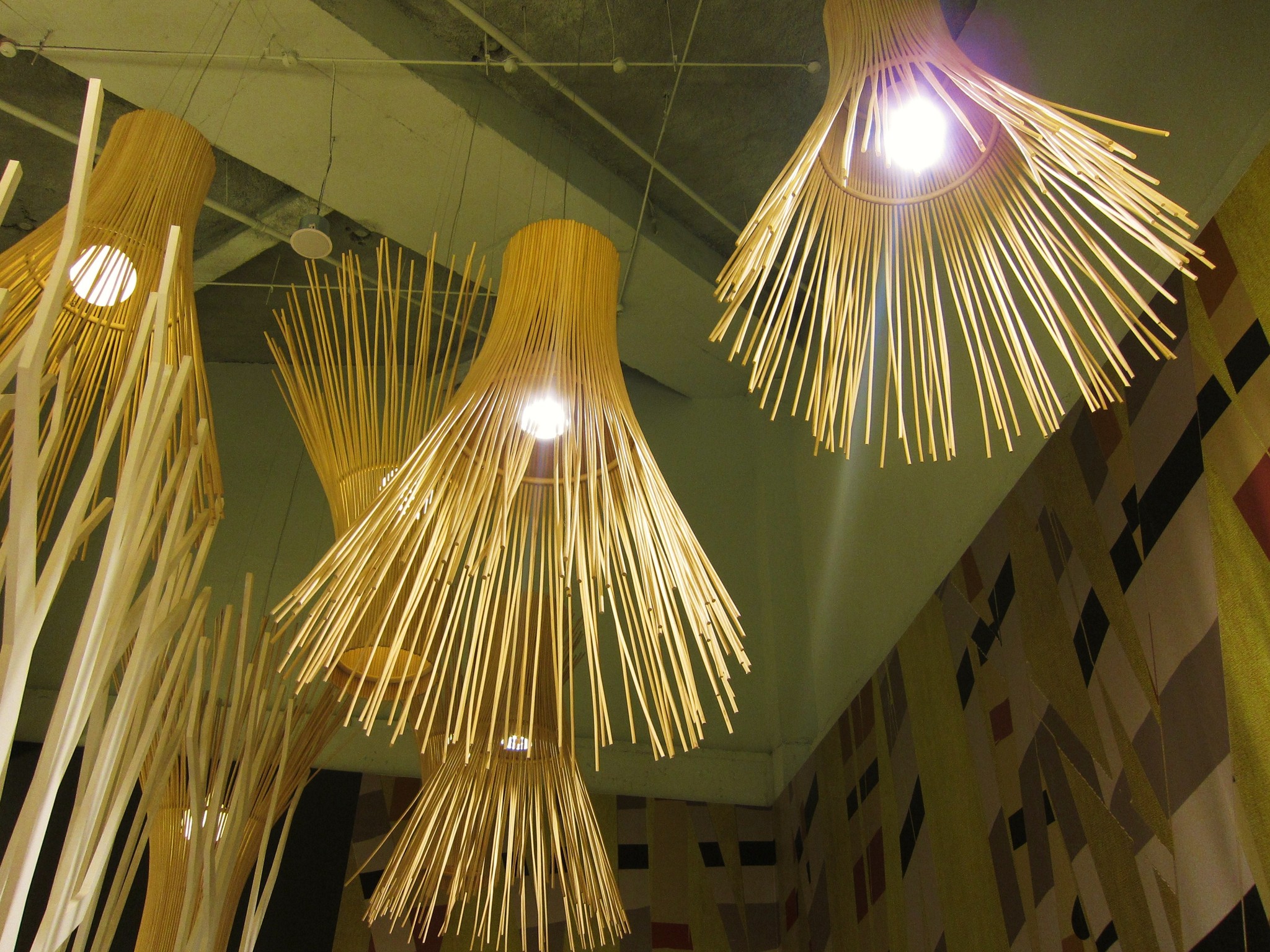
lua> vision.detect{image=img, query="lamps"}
[289,57,335,259]
[341,586,634,951]
[709,1,1214,466]
[259,1,502,714]
[0,1,256,566]
[268,1,746,761]
[112,446,359,952]
